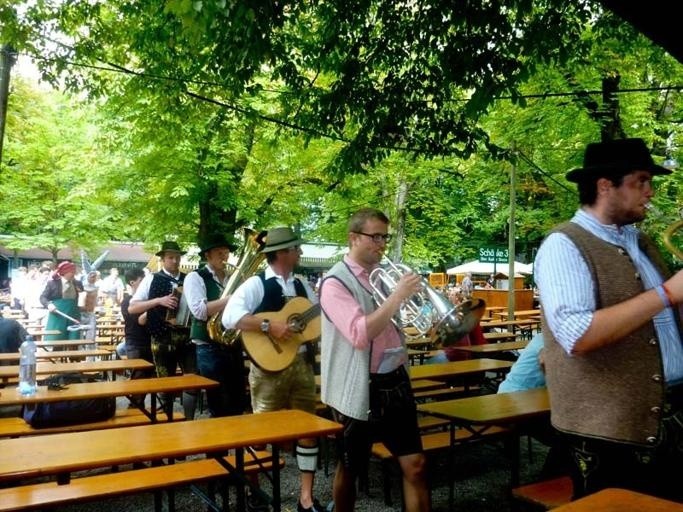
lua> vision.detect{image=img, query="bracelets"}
[654,286,670,308]
[660,284,674,306]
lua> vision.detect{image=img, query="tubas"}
[369,248,484,349]
[207,227,265,345]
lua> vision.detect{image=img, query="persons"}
[533,139,683,503]
[496,334,579,482]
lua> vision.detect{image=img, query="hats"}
[258,227,305,254]
[155,242,187,256]
[564,138,672,181]
[198,235,238,256]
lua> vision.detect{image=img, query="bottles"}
[17,335,40,396]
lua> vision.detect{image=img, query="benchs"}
[511,476,574,510]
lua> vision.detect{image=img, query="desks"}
[2,306,345,510]
[551,487,682,511]
[371,287,551,510]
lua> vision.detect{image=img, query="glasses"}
[351,231,392,244]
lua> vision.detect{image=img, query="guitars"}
[242,297,323,372]
